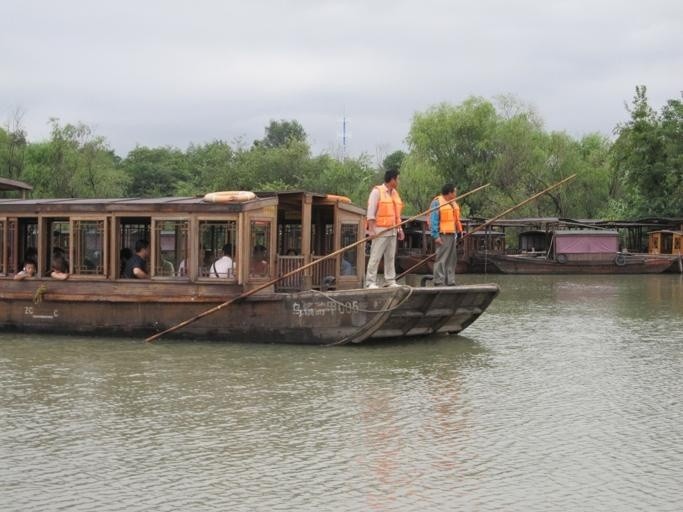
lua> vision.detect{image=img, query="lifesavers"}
[203,190,256,203]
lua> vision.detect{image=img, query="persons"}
[426,182,465,288]
[358,169,405,288]
[178,243,236,279]
[120,240,175,278]
[250,245,352,277]
[13,247,101,281]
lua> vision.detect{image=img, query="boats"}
[0,190,501,344]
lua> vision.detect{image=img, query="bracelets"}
[397,228,403,232]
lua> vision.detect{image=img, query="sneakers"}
[366,284,377,289]
[388,284,399,287]
